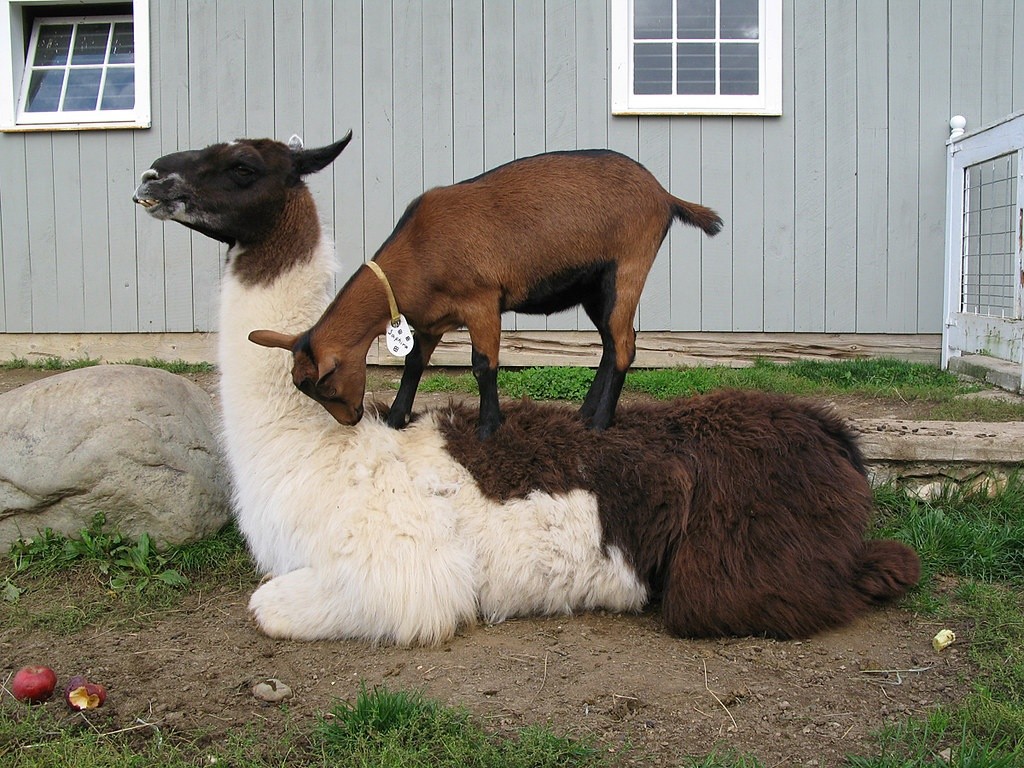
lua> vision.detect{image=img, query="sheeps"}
[248,149,726,437]
[133,129,921,646]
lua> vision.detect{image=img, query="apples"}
[65,677,105,710]
[13,666,57,704]
